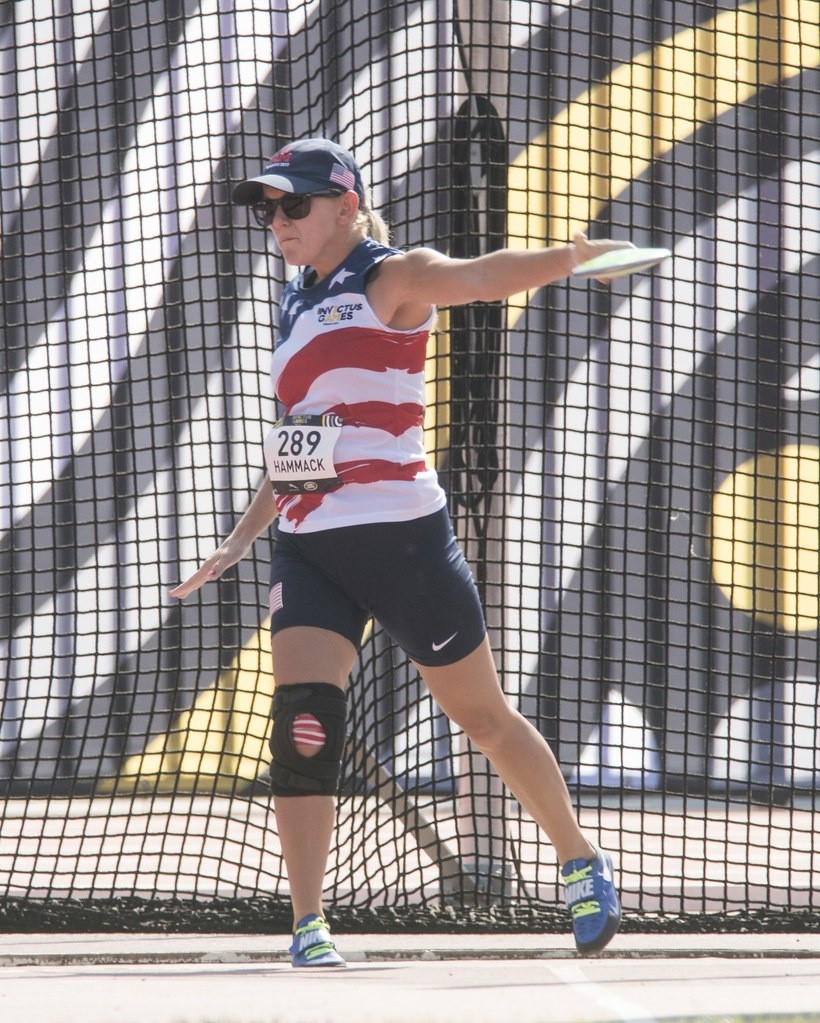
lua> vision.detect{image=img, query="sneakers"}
[290,913,348,968]
[560,837,621,955]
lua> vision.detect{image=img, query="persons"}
[169,137,638,968]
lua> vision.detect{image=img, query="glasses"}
[252,188,344,227]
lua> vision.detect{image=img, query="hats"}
[230,139,363,210]
